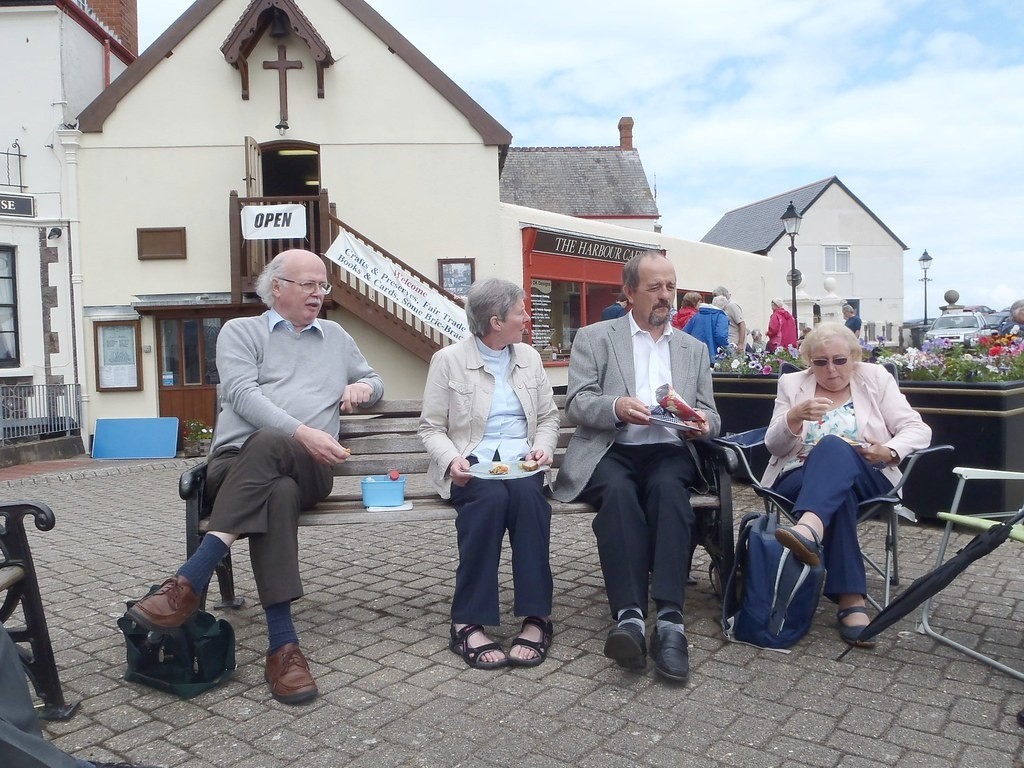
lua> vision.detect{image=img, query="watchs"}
[889,448,896,464]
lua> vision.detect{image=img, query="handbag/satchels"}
[117,585,237,700]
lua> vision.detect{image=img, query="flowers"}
[183,416,204,440]
[711,322,1024,384]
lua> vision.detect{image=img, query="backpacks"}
[720,513,826,651]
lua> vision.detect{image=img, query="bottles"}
[384,470,400,481]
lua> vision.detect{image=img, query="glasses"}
[274,275,333,297]
[809,356,849,366]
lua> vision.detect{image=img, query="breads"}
[522,460,537,471]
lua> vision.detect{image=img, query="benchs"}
[179,394,739,609]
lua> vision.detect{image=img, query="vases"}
[710,372,1024,535]
[184,440,212,456]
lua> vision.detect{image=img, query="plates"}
[648,416,702,432]
[800,440,868,448]
[463,460,543,480]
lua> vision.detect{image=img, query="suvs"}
[964,305,996,315]
[923,311,996,347]
[982,312,1011,331]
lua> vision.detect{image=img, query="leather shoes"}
[265,641,318,704]
[650,624,690,681]
[126,576,203,633]
[603,624,647,669]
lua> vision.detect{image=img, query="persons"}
[760,321,932,646]
[877,337,885,348]
[799,327,812,340]
[129,249,384,704]
[561,310,581,351]
[541,250,722,683]
[670,286,754,368]
[1002,307,1024,332]
[601,294,630,321]
[842,305,861,339]
[765,297,798,355]
[751,329,766,352]
[417,278,561,670]
[0,622,161,768]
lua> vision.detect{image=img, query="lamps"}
[48,228,62,240]
[275,115,289,137]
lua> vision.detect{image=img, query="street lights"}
[780,200,804,341]
[917,248,933,326]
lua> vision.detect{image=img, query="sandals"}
[508,617,553,666]
[448,621,507,669]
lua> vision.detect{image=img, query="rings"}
[627,409,634,415]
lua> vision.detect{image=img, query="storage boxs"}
[720,427,772,485]
[361,475,405,507]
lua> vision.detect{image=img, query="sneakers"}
[775,523,823,566]
[837,606,877,645]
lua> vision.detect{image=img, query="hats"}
[772,298,783,307]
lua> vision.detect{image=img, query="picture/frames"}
[438,257,476,288]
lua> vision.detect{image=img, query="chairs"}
[0,501,82,719]
[922,467,1024,681]
[712,361,956,612]
